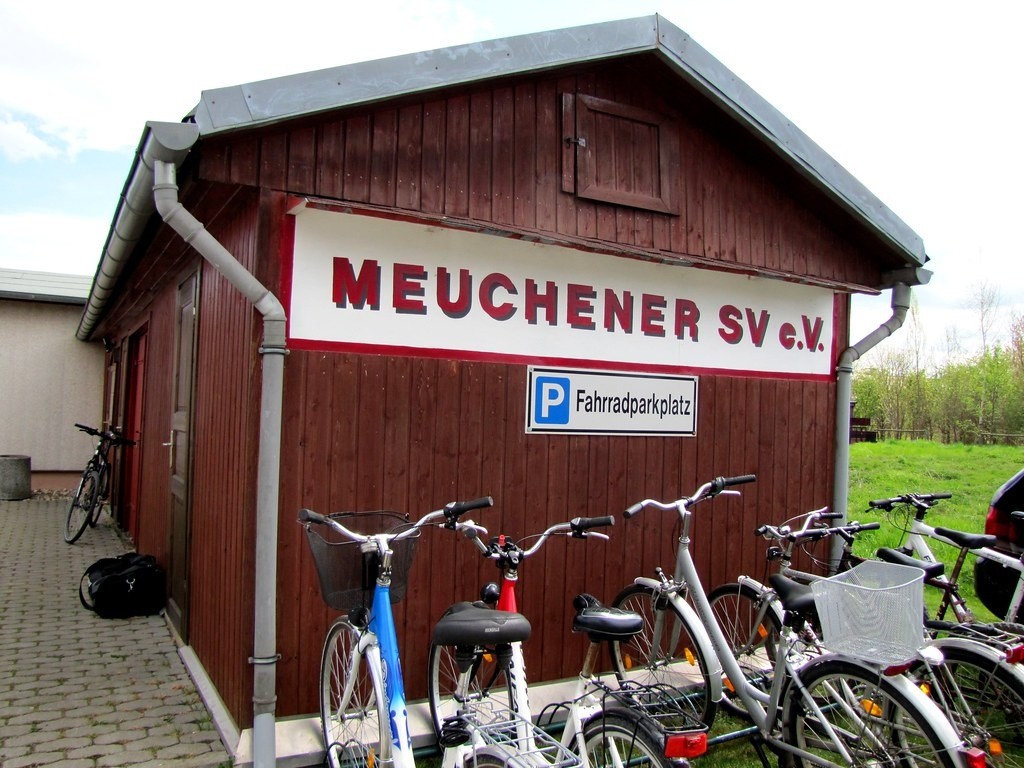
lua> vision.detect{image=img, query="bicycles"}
[296,493,581,768]
[63,422,138,545]
[426,513,710,768]
[705,488,1023,767]
[607,473,988,768]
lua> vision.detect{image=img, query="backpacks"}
[78,553,165,617]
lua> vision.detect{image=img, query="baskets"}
[303,512,420,611]
[810,561,925,666]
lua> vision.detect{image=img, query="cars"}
[974,466,1024,627]
